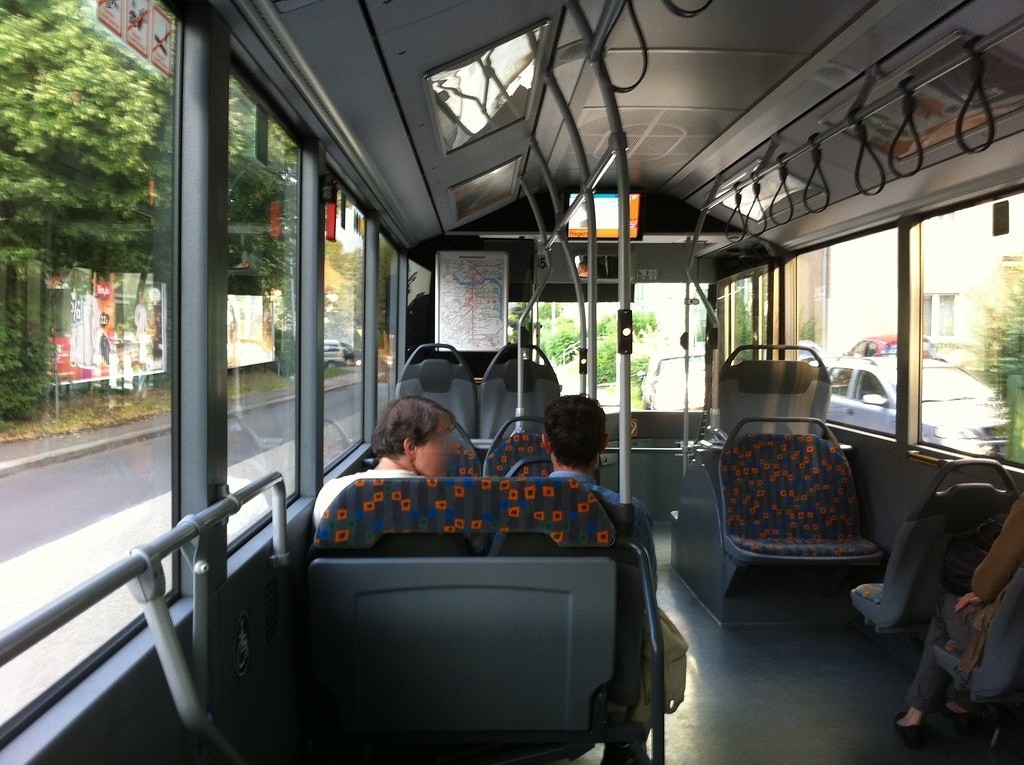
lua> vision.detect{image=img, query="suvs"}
[819,356,1013,460]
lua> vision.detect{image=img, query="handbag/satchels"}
[940,518,1002,595]
[642,603,689,715]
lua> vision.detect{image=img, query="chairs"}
[697,345,832,449]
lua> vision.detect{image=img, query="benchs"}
[720,418,884,570]
[851,458,1023,708]
[303,343,670,765]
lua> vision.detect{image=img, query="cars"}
[323,340,354,370]
[638,352,706,411]
[797,340,825,363]
[834,335,940,371]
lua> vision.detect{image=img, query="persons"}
[541,393,658,594]
[311,398,462,537]
[894,490,1024,749]
[578,262,588,278]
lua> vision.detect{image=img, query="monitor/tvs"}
[567,193,644,242]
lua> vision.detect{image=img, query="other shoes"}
[600,744,637,765]
[894,712,923,749]
[940,701,975,735]
